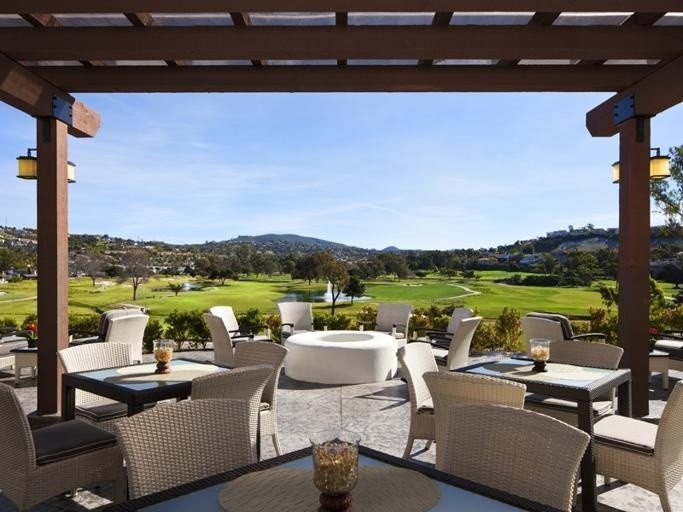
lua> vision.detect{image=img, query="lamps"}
[15,147,36,182]
[65,158,78,186]
[648,145,672,182]
[611,157,621,185]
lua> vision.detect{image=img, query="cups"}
[529,337,550,361]
[309,428,362,497]
[151,338,173,363]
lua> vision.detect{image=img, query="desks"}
[64,437,562,512]
[59,353,234,423]
[447,355,634,512]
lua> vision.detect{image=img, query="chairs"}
[416,368,526,423]
[434,400,594,512]
[232,338,291,460]
[199,298,486,376]
[523,338,625,488]
[393,342,443,462]
[188,363,276,463]
[521,292,682,424]
[53,340,156,432]
[0,303,150,388]
[0,381,118,512]
[112,396,252,499]
[592,377,683,512]
